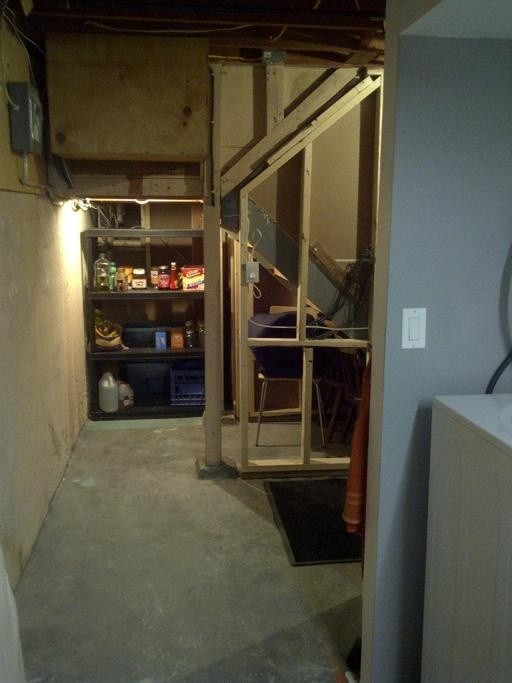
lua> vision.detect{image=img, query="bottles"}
[93,254,109,289]
[159,266,170,288]
[116,263,132,290]
[184,321,196,347]
[110,262,116,289]
[170,262,179,289]
[119,380,135,408]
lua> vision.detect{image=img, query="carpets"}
[264,478,361,567]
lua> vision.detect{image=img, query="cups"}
[149,267,159,286]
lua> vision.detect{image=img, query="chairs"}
[249,309,339,449]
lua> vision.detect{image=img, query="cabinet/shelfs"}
[85,229,206,422]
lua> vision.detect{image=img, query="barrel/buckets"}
[98,371,119,413]
[93,252,112,291]
[117,381,136,409]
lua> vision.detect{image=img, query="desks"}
[421,394,511,683]
